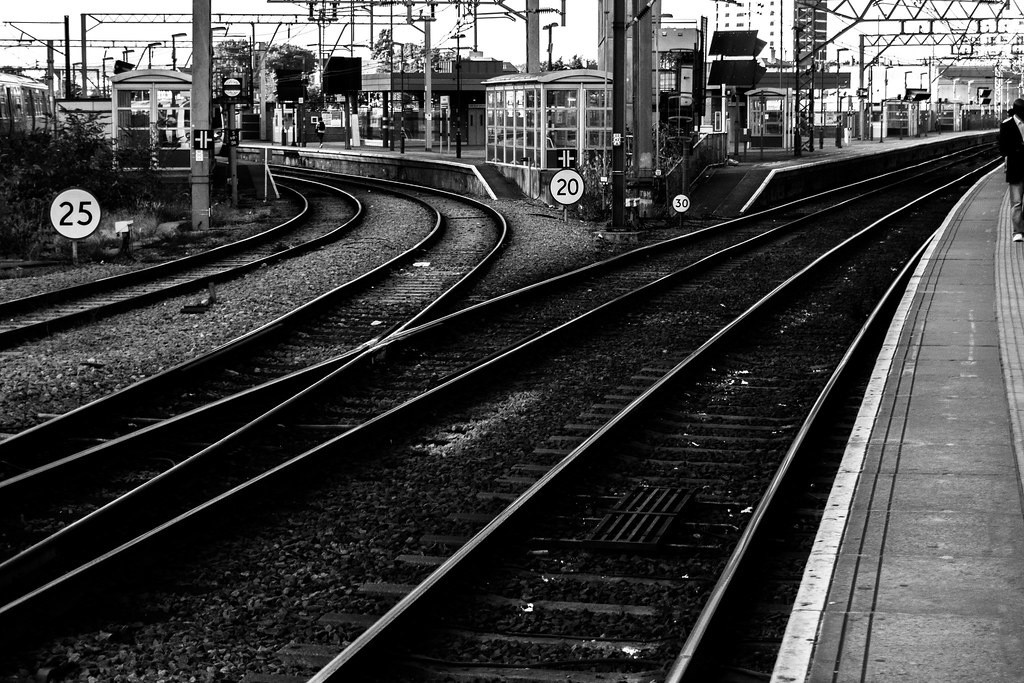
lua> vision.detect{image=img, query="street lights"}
[72,62,83,97]
[148,43,161,69]
[172,33,188,70]
[102,57,114,98]
[123,50,135,61]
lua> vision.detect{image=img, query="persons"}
[1002,109,1014,173]
[315,117,325,148]
[1001,98,1024,241]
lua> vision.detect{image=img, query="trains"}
[0,72,53,136]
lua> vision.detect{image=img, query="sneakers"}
[1011,234,1024,242]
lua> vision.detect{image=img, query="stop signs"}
[222,78,243,99]
